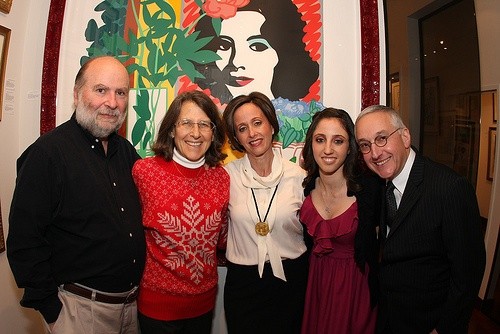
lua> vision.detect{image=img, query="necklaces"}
[318,179,330,211]
[250,185,279,237]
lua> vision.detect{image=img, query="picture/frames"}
[422,77,440,133]
[487,127,497,180]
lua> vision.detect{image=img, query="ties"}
[384,180,398,230]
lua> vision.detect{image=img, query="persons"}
[221,91,308,334]
[348,102,500,334]
[132,89,231,334]
[7,56,147,334]
[298,108,377,334]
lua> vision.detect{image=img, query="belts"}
[63,284,139,305]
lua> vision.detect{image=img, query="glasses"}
[359,128,400,154]
[175,118,216,132]
